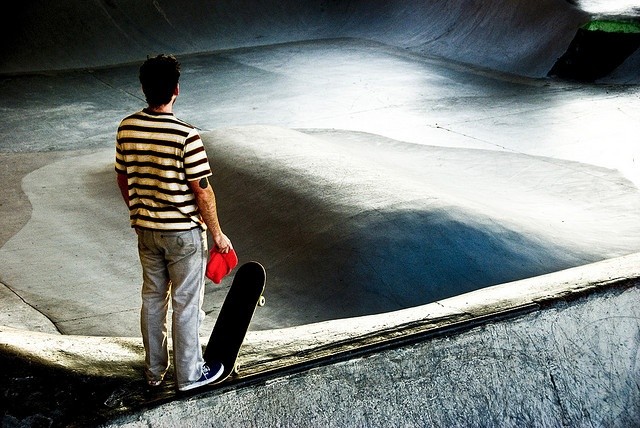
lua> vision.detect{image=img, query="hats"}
[205,242,238,284]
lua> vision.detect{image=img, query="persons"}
[114,52,233,394]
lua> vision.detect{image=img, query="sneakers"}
[177,360,225,390]
[148,365,170,386]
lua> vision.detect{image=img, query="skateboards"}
[203,262,267,386]
